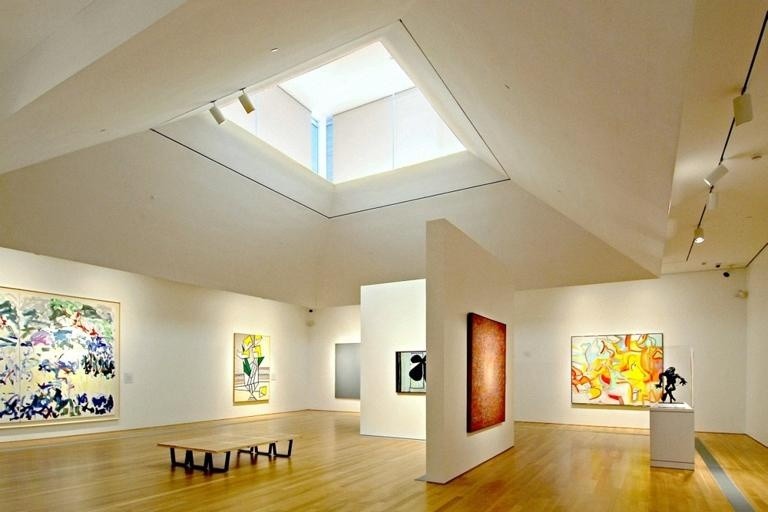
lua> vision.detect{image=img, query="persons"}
[655,367,688,401]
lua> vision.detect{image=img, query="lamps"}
[208,99,225,124]
[237,87,256,114]
[686,10,768,260]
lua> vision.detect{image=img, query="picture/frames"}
[0,285,122,429]
[467,311,506,433]
[395,351,427,393]
[571,333,664,407]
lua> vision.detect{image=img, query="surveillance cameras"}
[309,309,313,312]
[723,272,729,277]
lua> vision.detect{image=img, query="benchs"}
[157,433,296,476]
